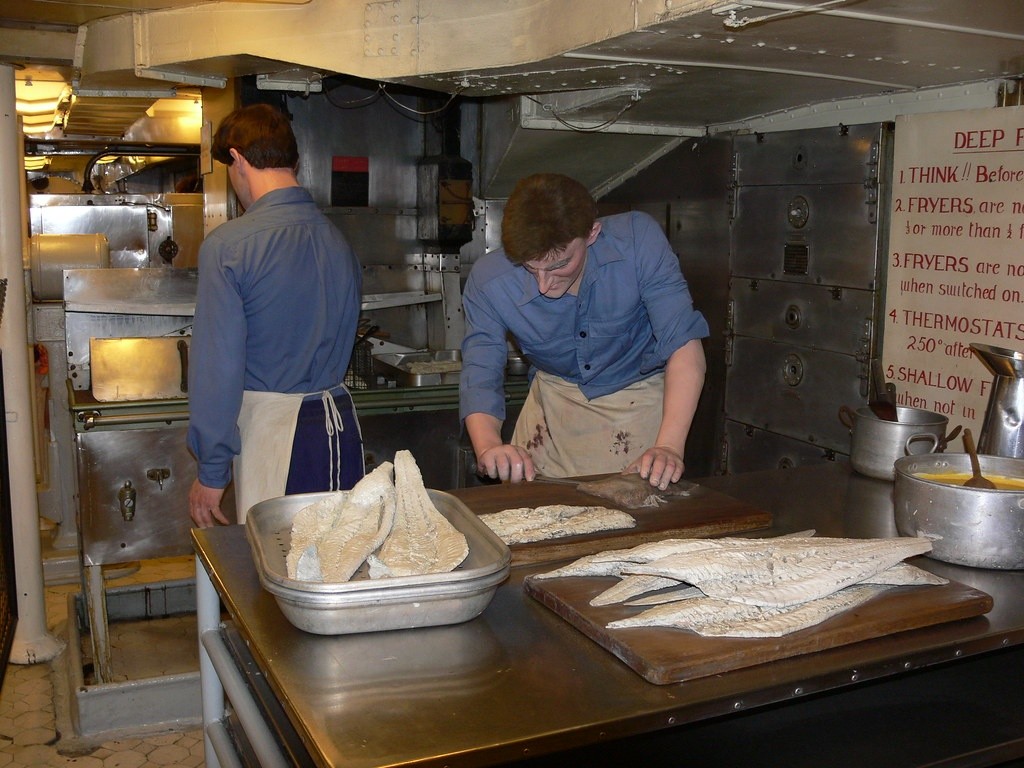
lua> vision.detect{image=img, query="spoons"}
[886,382,897,422]
[871,357,898,423]
[964,428,997,491]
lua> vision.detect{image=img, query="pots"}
[892,433,1024,571]
[837,402,962,482]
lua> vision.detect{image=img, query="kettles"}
[968,340,1024,460]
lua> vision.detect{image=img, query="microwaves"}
[61,311,196,409]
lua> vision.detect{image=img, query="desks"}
[188,458,1024,767]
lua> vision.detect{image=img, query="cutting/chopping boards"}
[444,470,771,567]
[521,563,992,688]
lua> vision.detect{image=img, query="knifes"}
[481,466,585,486]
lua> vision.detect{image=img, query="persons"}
[185,102,369,527]
[456,173,710,490]
